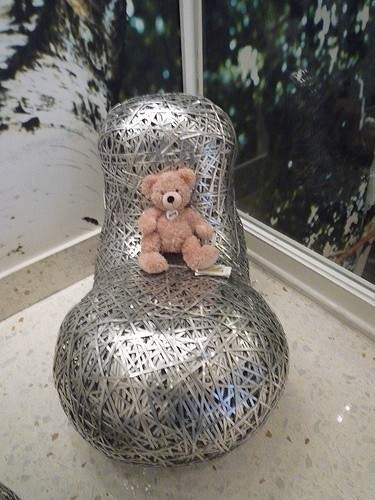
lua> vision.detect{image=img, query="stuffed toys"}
[135,166,219,276]
